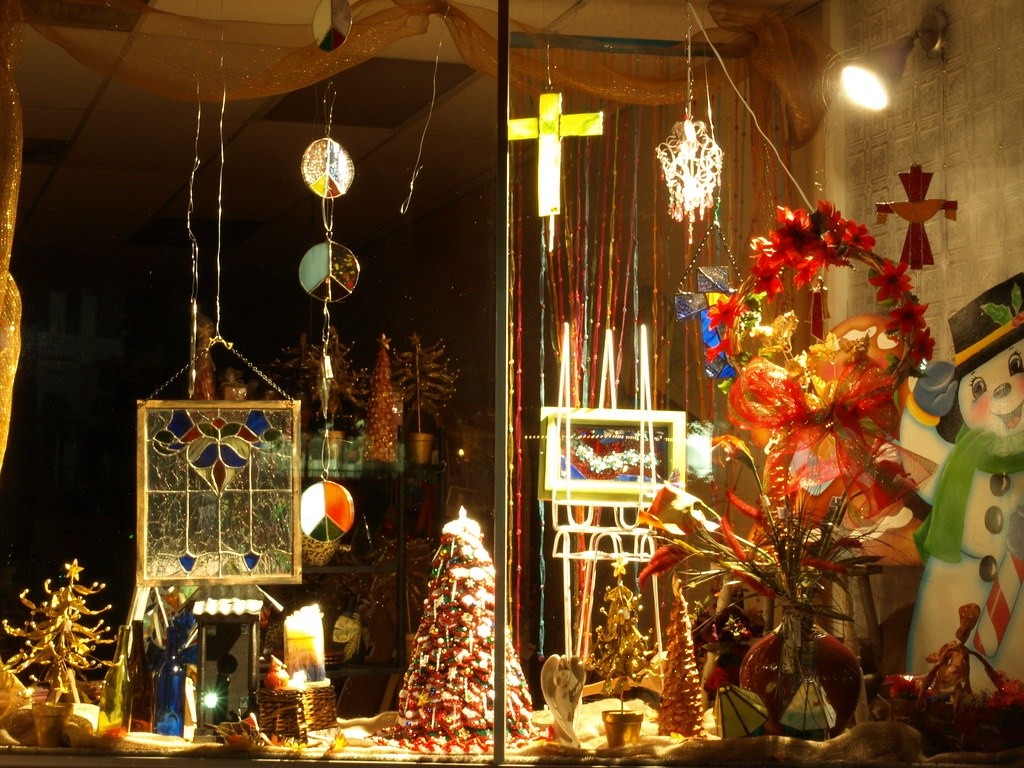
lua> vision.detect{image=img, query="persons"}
[148,585,199,741]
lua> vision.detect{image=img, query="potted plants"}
[587,574,671,749]
[7,562,126,750]
[300,325,371,474]
[392,332,461,472]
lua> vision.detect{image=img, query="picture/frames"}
[538,406,686,509]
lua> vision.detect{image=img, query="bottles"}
[265,657,289,691]
[129,619,155,732]
[98,625,130,734]
[157,627,185,736]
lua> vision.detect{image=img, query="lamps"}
[654,0,725,248]
[839,8,947,112]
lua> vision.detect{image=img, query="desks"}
[700,515,928,729]
